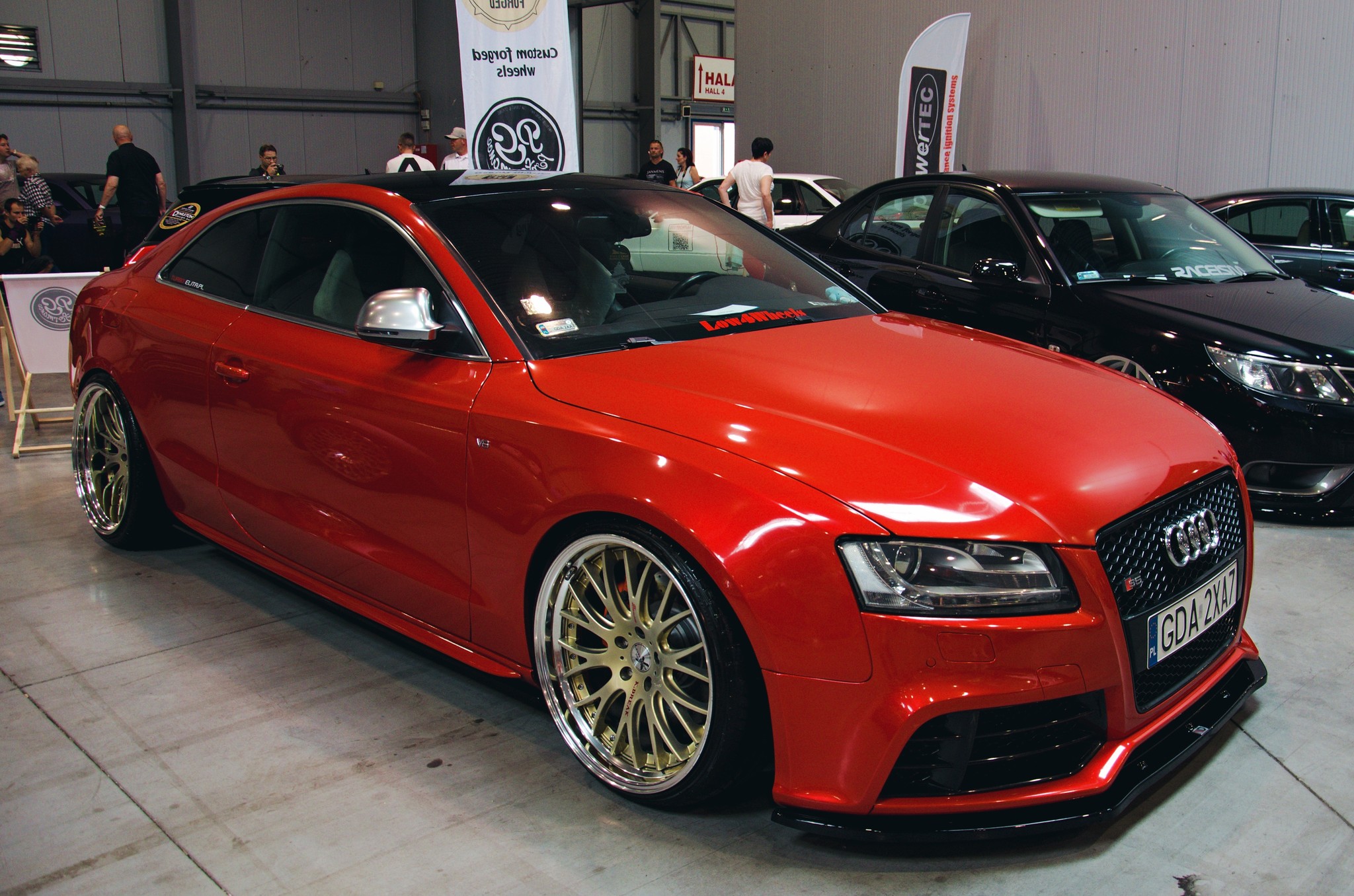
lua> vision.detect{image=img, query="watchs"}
[98,207,100,209]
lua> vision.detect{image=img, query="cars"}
[775,171,1354,520]
[64,171,1271,833]
[1092,186,1354,293]
[687,172,861,230]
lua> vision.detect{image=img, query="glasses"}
[263,157,277,161]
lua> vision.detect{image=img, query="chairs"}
[1297,222,1312,245]
[1050,218,1105,277]
[947,208,1027,275]
[311,252,368,327]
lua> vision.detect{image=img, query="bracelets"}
[263,174,265,176]
[99,205,105,209]
[33,232,40,237]
[266,171,270,176]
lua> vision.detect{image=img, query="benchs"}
[869,221,922,256]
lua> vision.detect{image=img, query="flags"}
[895,13,971,210]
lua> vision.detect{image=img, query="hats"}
[444,127,466,139]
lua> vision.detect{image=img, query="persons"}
[639,140,678,187]
[13,149,63,254]
[0,133,40,218]
[386,132,436,174]
[0,198,44,309]
[718,137,774,229]
[675,148,700,189]
[440,127,489,170]
[94,125,166,252]
[249,145,286,177]
